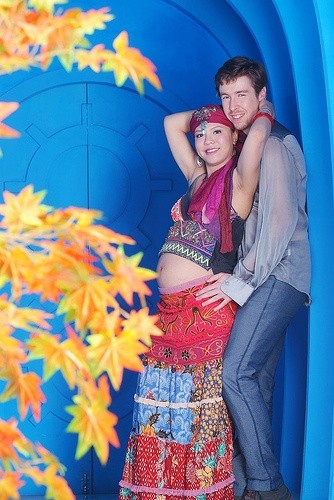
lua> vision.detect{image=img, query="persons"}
[195,56,312,500]
[118,101,275,500]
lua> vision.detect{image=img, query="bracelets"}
[251,102,274,128]
